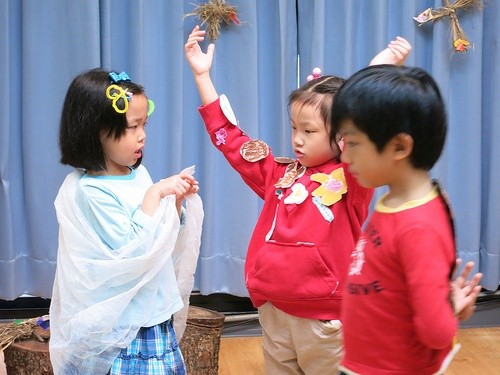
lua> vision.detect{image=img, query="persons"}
[329,64,482,375]
[49,70,204,375]
[184,25,412,375]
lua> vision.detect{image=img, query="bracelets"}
[158,189,165,200]
